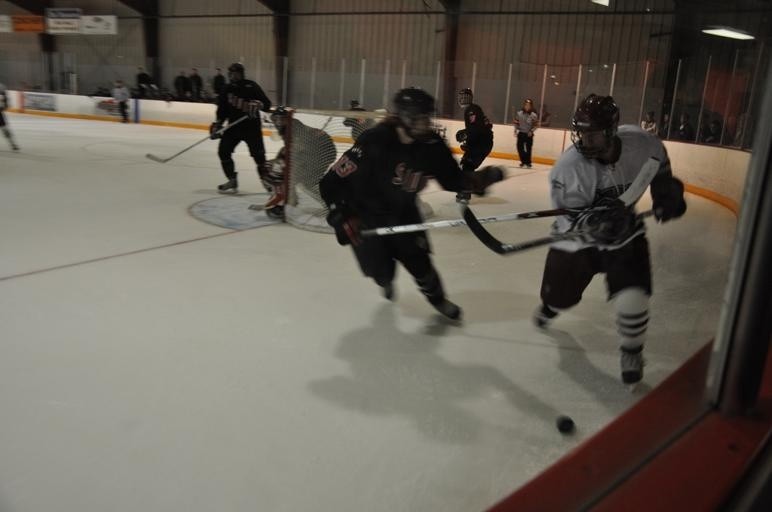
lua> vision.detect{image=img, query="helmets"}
[389,87,434,141]
[570,94,620,159]
[457,87,473,108]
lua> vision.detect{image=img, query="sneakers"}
[262,192,285,209]
[621,345,643,383]
[218,173,237,190]
[535,302,559,329]
[428,294,464,322]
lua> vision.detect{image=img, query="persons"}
[20,80,32,91]
[536,104,551,126]
[249,107,336,218]
[319,86,503,319]
[509,105,517,123]
[513,97,538,167]
[33,81,42,91]
[0,91,19,151]
[85,67,226,123]
[343,100,376,139]
[532,93,686,383]
[456,88,494,200]
[210,62,272,192]
[641,110,722,146]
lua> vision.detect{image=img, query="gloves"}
[569,196,637,244]
[456,129,470,142]
[209,122,224,140]
[244,100,265,118]
[650,174,687,224]
